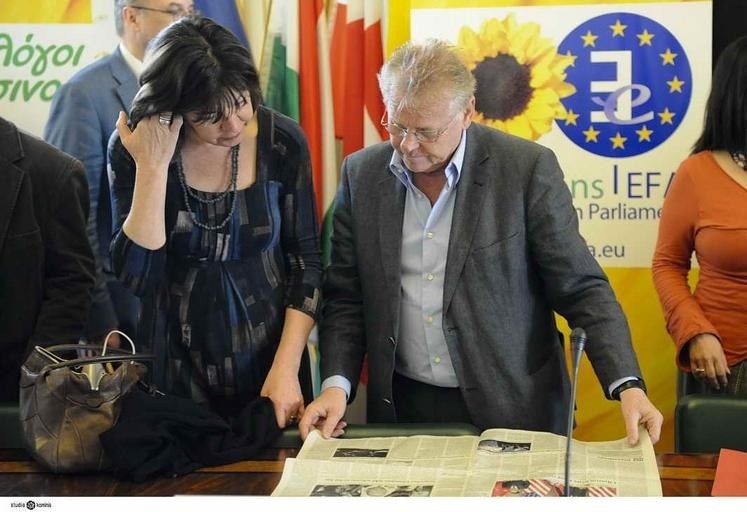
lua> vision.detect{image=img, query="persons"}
[298,37,666,450]
[651,35,747,398]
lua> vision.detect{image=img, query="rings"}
[696,368,705,372]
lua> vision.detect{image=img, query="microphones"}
[563,327,588,497]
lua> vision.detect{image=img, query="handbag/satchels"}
[17,342,158,478]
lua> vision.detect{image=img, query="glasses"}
[379,107,463,145]
[127,4,201,23]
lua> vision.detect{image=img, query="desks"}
[4,444,743,498]
